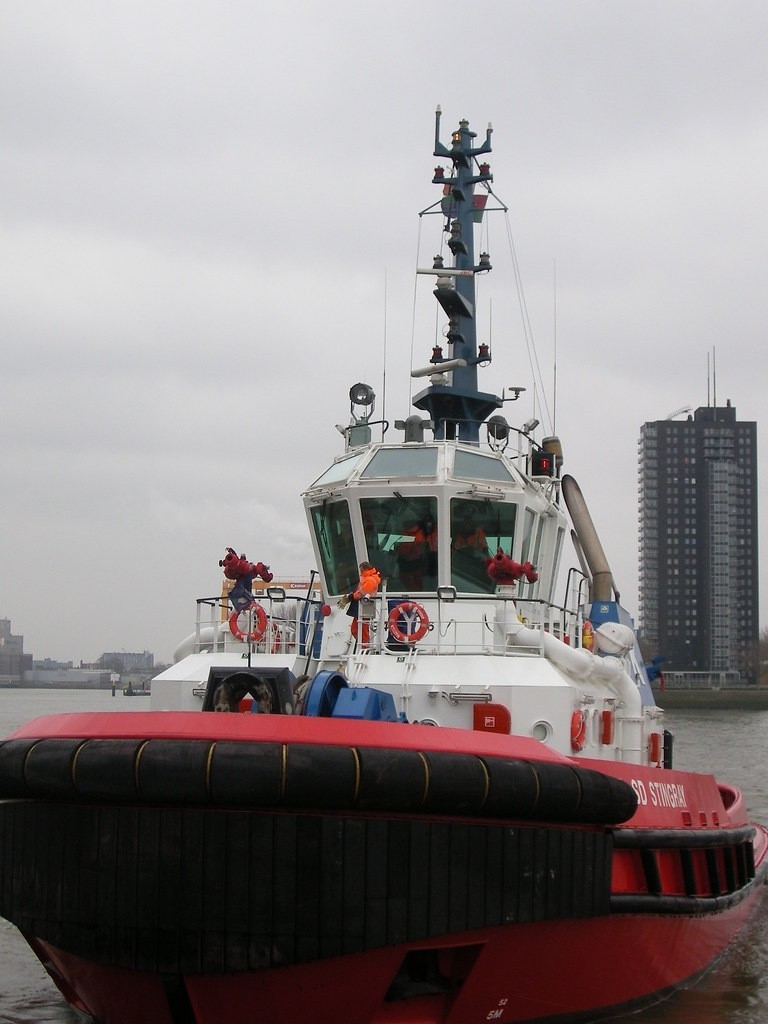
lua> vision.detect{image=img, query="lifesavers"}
[258,622,280,653]
[584,619,597,652]
[387,604,430,644]
[228,602,270,643]
[571,711,588,751]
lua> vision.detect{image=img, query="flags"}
[472,194,488,223]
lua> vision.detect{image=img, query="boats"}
[0,100,768,1023]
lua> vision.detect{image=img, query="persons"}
[415,514,438,551]
[451,517,488,554]
[337,562,381,655]
